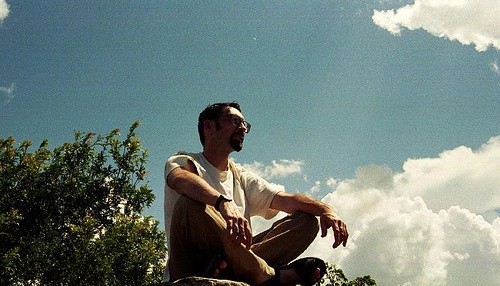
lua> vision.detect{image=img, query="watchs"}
[213,194,233,212]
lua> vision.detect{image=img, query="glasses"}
[213,115,251,133]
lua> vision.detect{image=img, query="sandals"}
[262,257,326,286]
[200,253,236,280]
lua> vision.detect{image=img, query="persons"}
[162,100,349,286]
